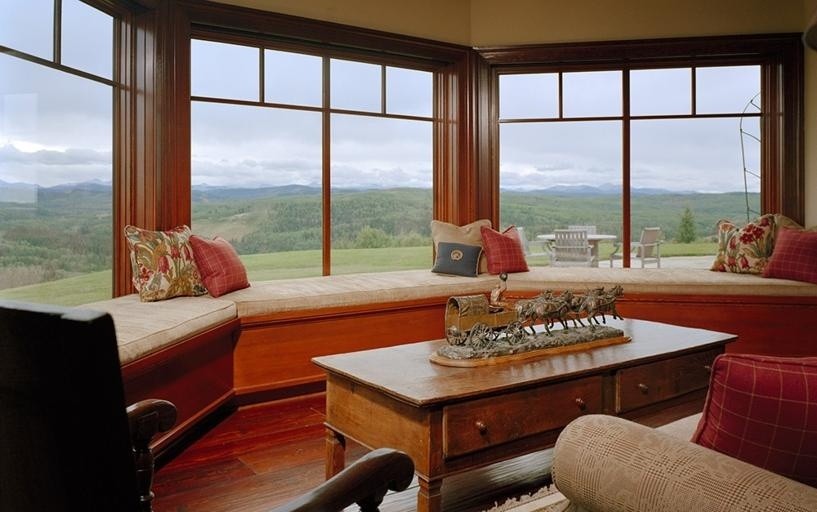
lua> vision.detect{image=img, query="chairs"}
[0,300,415,512]
[516,225,663,269]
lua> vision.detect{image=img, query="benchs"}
[500,266,817,357]
[219,269,500,396]
[73,293,240,460]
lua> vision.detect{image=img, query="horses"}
[514,285,624,337]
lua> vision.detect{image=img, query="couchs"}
[551,410,817,512]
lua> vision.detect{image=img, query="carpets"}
[483,484,571,512]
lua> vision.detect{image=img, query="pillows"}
[124,224,208,302]
[689,353,817,487]
[189,235,251,298]
[430,219,530,277]
[710,213,817,284]
